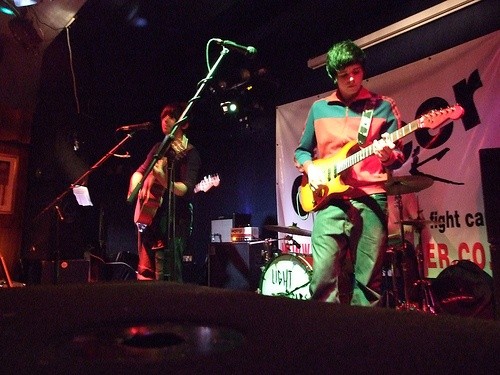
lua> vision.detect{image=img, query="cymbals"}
[392,217,446,228]
[383,175,434,195]
[265,224,309,236]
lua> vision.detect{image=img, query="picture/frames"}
[0,153,20,214]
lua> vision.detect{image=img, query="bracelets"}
[380,158,389,163]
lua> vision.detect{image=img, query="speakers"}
[209,242,274,294]
[479,147,500,323]
[39,259,91,285]
[211,213,251,243]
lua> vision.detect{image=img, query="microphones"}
[293,240,300,249]
[213,38,257,56]
[120,121,152,131]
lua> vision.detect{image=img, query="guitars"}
[193,173,221,194]
[299,102,465,213]
[132,133,190,226]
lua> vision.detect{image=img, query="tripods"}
[380,194,421,312]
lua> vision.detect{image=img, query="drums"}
[258,252,314,300]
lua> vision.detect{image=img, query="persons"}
[381,168,425,310]
[293,41,405,307]
[126,99,202,283]
